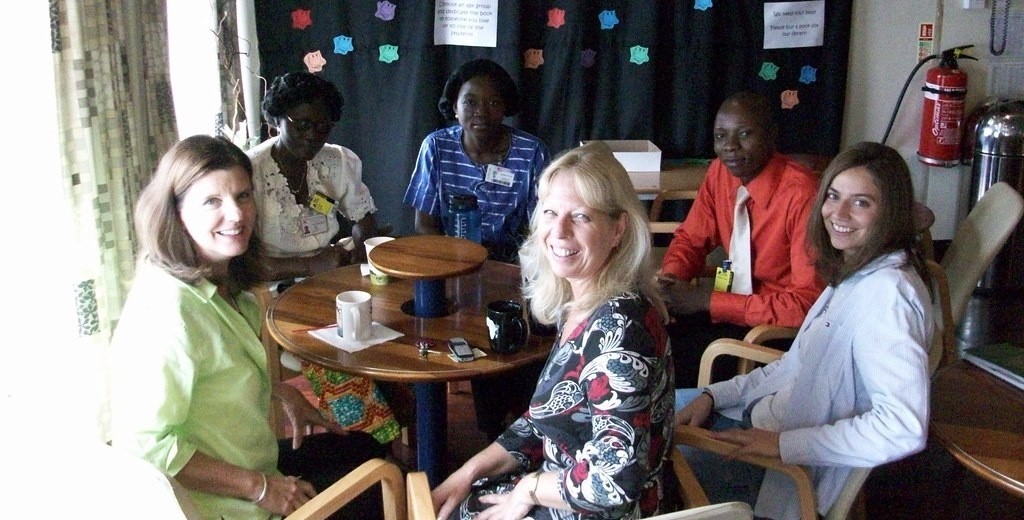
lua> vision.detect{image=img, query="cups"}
[527,299,558,335]
[484,302,529,353]
[448,194,481,248]
[336,290,372,342]
[363,237,397,286]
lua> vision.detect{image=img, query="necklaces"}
[274,147,305,194]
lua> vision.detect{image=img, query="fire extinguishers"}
[881,45,979,168]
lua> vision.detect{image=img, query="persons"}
[241,71,417,461]
[653,88,823,388]
[431,138,676,520]
[664,140,936,520]
[110,132,347,520]
[402,58,551,442]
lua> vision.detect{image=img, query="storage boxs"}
[578,139,662,172]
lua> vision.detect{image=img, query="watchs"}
[527,470,547,506]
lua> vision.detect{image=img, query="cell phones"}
[447,337,475,362]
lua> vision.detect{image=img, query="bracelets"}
[251,471,267,505]
[702,390,714,408]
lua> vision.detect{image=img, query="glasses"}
[285,114,335,134]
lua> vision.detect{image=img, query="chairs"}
[154,183,1024,520]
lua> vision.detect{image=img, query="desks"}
[622,166,710,237]
[266,235,560,484]
[931,355,1024,494]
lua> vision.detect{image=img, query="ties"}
[728,185,753,295]
